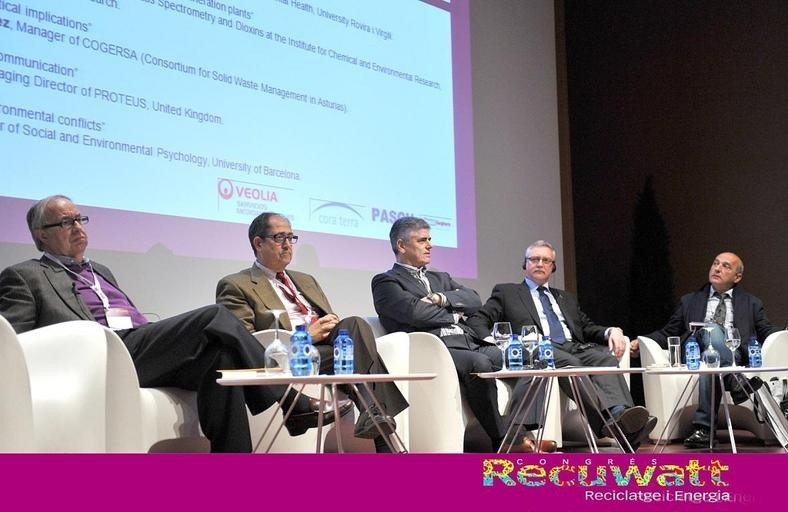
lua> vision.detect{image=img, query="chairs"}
[245,329,410,455]
[557,333,631,448]
[0,313,109,455]
[103,320,306,453]
[638,330,788,444]
[363,312,558,454]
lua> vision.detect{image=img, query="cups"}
[667,336,682,369]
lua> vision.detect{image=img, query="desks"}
[641,365,788,454]
[472,367,645,454]
[211,374,436,453]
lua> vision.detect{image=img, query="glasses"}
[258,233,298,245]
[41,216,89,228]
[525,257,553,264]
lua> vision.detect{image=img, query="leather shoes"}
[497,435,541,453]
[354,404,397,440]
[685,428,718,449]
[527,439,557,453]
[732,375,764,405]
[601,405,650,439]
[622,415,659,453]
[282,395,354,437]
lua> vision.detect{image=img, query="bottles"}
[684,337,700,370]
[537,335,555,368]
[333,328,354,375]
[748,334,762,368]
[289,317,313,376]
[508,334,524,370]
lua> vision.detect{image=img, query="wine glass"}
[493,321,513,372]
[263,308,291,377]
[702,325,721,368]
[723,327,744,369]
[520,325,539,370]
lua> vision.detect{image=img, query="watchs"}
[426,292,437,304]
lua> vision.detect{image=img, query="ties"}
[536,286,566,345]
[713,292,730,326]
[277,271,320,323]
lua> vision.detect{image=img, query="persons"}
[1,193,357,453]
[628,251,786,448]
[465,238,659,454]
[215,211,410,453]
[371,216,559,453]
